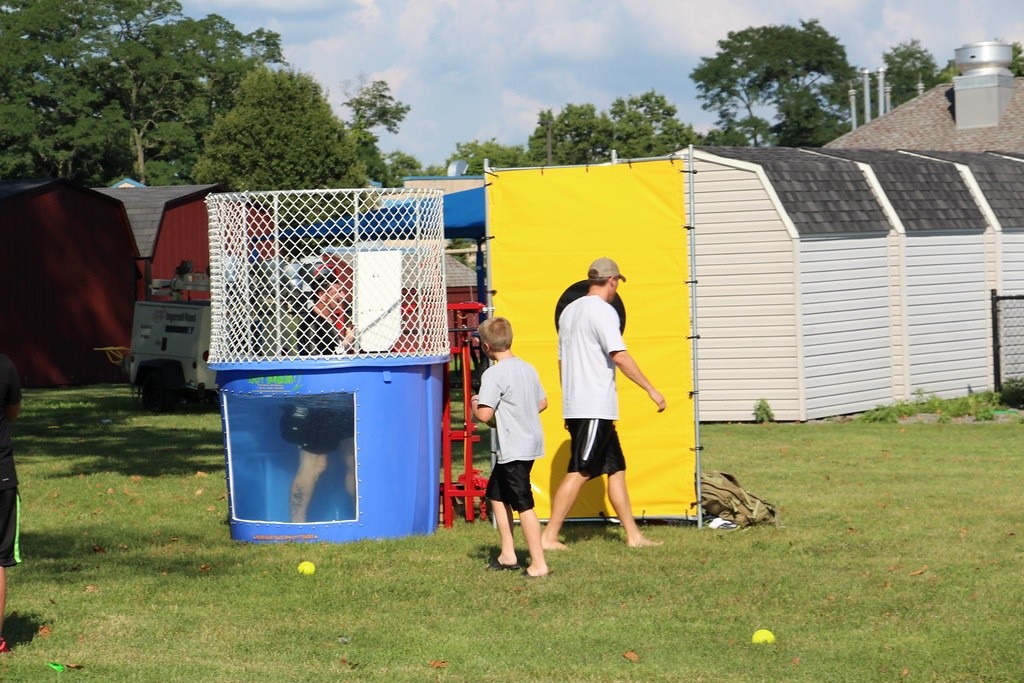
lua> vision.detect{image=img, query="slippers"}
[522,569,531,578]
[485,559,520,571]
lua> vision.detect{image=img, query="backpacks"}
[696,468,776,528]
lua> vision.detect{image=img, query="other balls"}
[752,629,775,645]
[298,561,316,576]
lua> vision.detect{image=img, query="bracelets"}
[473,399,478,401]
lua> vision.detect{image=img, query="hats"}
[588,258,626,282]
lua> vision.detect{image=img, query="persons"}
[289,274,356,355]
[0,352,23,654]
[542,256,667,550]
[471,316,554,579]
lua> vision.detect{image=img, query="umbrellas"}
[249,186,487,324]
